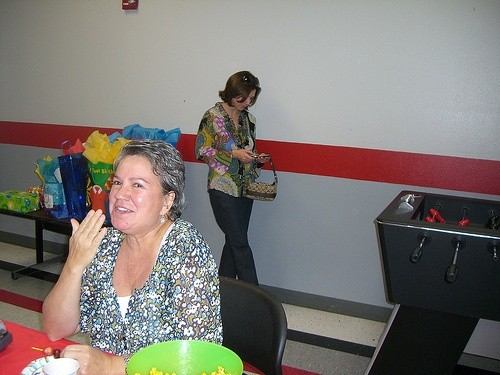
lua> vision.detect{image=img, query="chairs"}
[216,276,288,375]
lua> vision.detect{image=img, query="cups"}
[43,358,80,374]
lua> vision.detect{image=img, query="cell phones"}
[251,155,269,158]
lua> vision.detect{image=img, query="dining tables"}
[0,319,118,375]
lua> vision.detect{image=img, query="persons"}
[42,138,223,375]
[195,70,271,287]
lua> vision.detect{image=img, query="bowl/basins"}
[127,340,243,375]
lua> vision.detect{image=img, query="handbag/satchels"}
[242,151,278,202]
[41,152,117,224]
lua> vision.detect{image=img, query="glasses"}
[241,76,261,87]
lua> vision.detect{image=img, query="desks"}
[0,208,113,279]
